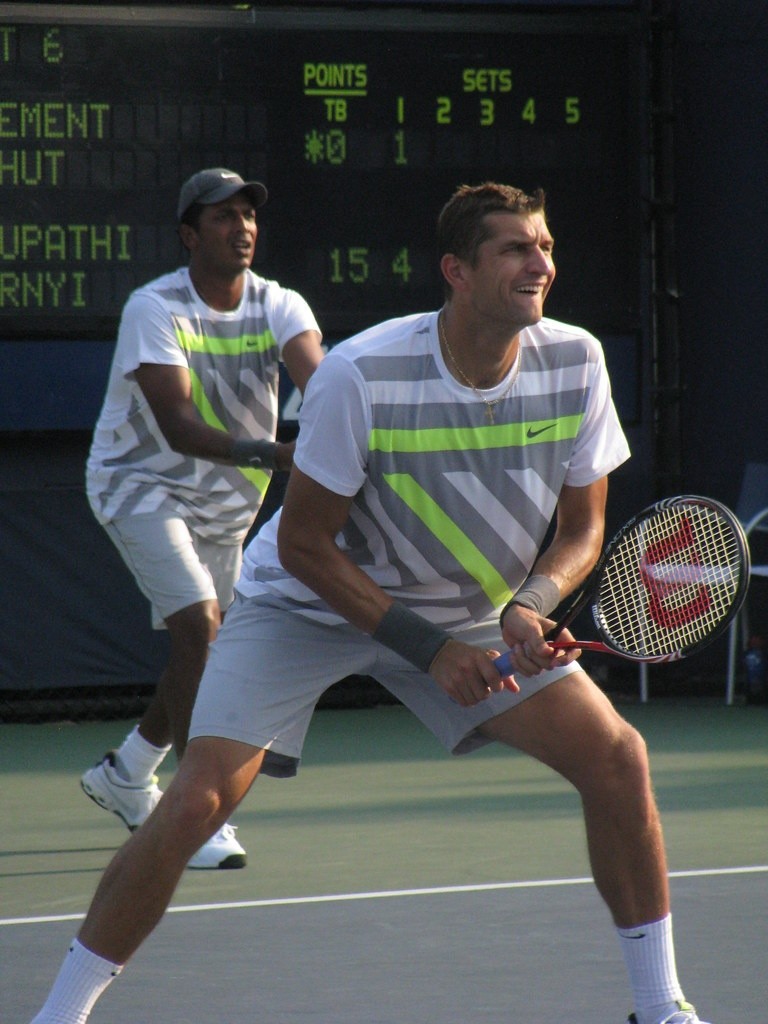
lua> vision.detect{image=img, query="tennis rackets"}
[447,493,751,706]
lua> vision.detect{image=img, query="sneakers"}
[186,822,247,869]
[80,752,164,836]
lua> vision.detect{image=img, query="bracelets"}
[230,439,282,472]
[372,600,453,673]
[499,574,560,631]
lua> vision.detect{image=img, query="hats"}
[176,167,269,224]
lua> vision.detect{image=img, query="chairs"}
[640,462,768,705]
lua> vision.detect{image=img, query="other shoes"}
[626,1000,711,1024]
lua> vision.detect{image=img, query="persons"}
[80,167,325,871]
[29,182,707,1023]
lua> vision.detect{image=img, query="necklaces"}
[439,315,521,426]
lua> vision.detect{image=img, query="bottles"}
[746,638,768,707]
